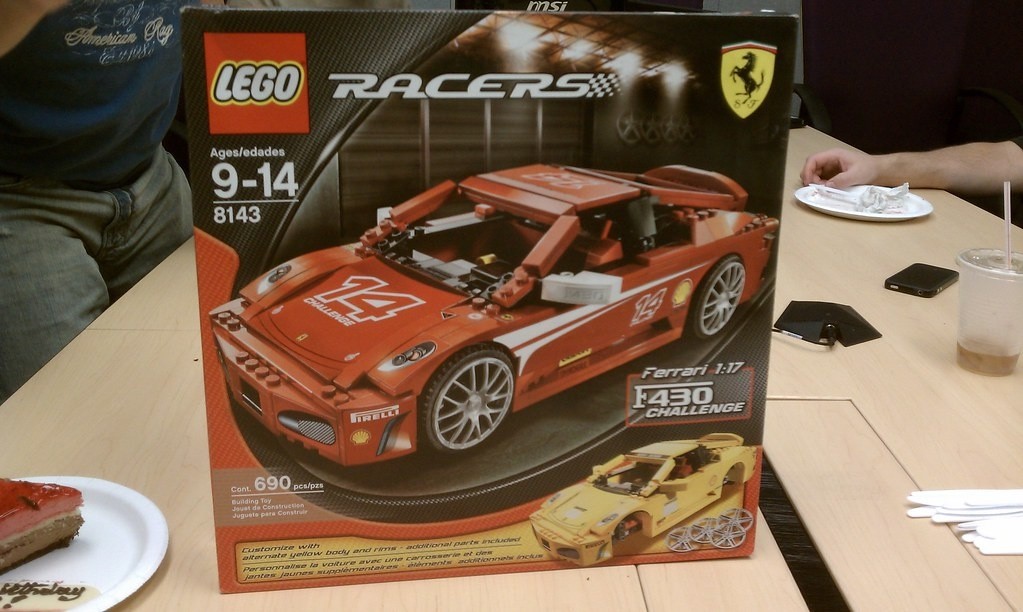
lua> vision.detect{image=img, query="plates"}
[795,184,934,223]
[0,476,168,612]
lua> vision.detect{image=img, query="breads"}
[0,582,103,612]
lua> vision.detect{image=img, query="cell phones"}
[886,263,959,298]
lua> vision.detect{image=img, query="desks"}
[0,230,808,612]
[761,125,1023,612]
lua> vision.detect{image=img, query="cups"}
[954,248,1023,379]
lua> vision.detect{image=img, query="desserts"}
[0,477,86,573]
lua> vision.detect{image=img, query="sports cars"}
[519,433,758,570]
[219,161,776,480]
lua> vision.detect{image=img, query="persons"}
[800,134,1023,191]
[0,0,194,395]
